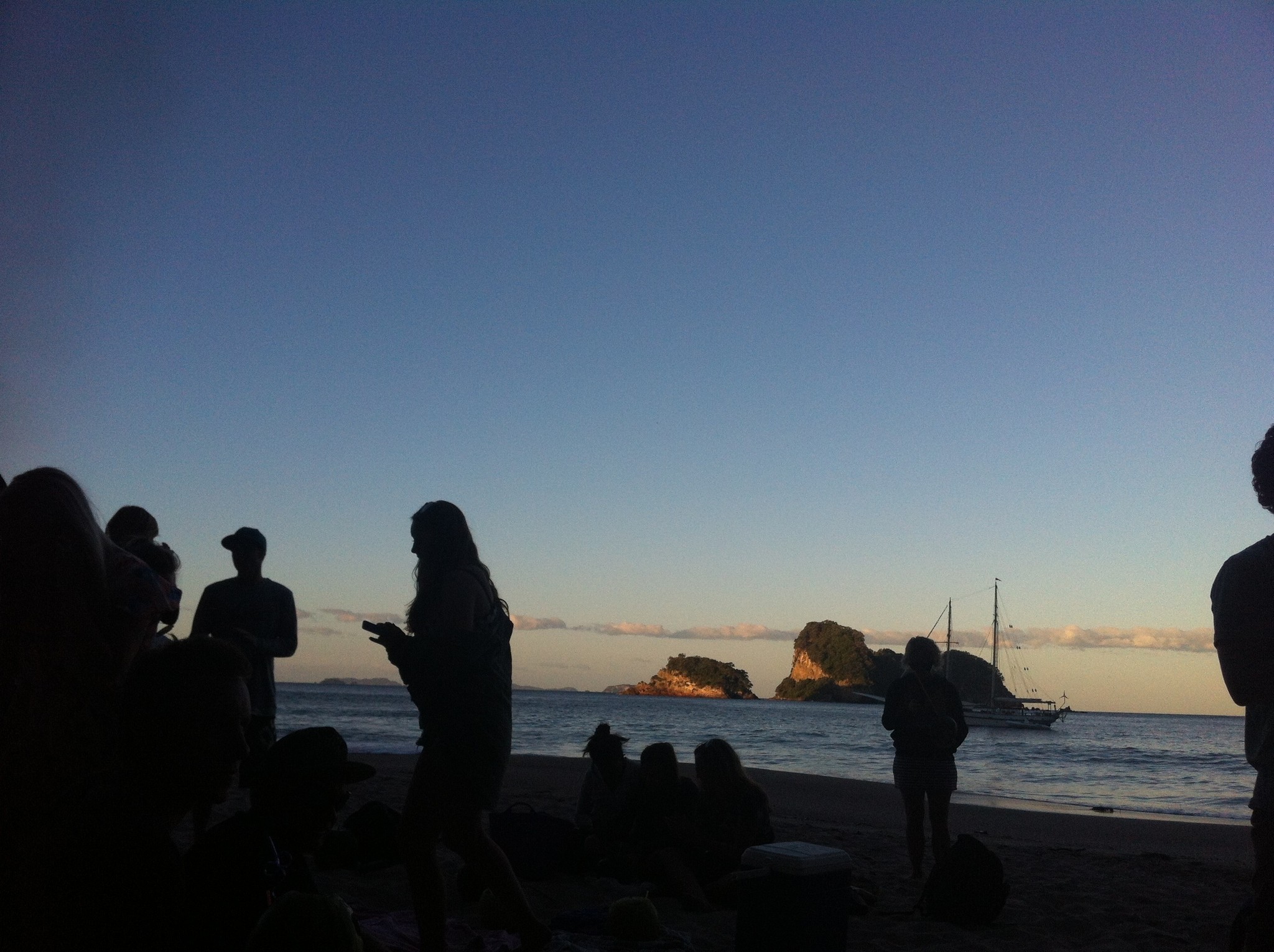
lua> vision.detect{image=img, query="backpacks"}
[918,832,1009,932]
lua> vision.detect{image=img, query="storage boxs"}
[731,838,854,952]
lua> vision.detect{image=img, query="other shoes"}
[516,923,553,952]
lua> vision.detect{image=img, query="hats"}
[222,528,266,553]
[261,727,376,794]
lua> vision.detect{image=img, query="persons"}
[364,501,559,952]
[1210,424,1274,952]
[661,738,779,915]
[589,741,700,905]
[574,723,647,886]
[0,465,407,952]
[882,637,969,885]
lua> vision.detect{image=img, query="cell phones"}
[361,621,381,635]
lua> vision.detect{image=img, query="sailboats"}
[851,575,1069,731]
